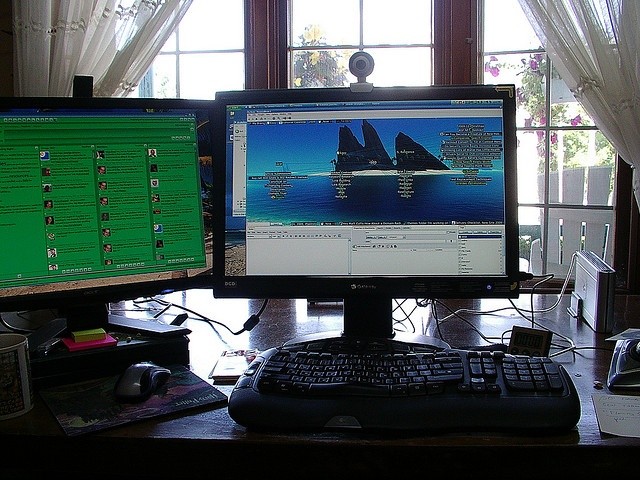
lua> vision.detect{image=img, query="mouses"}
[112,361,172,402]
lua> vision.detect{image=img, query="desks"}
[0,289,639,480]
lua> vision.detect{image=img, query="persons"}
[47,216,52,224]
[99,166,105,173]
[102,228,110,237]
[101,214,108,221]
[100,182,106,189]
[151,164,157,171]
[157,240,163,248]
[46,200,52,208]
[45,168,50,176]
[106,260,112,265]
[100,197,108,205]
[149,150,156,157]
[104,244,112,253]
[49,249,56,257]
[44,185,52,192]
[50,265,56,270]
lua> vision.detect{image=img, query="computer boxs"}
[1,311,188,388]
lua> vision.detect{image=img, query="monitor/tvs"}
[1,96,202,336]
[213,83,519,349]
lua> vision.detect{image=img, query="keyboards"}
[228,349,581,432]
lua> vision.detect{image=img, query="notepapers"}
[62,333,117,352]
[72,328,106,343]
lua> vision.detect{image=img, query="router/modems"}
[573,249,616,335]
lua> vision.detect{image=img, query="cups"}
[0,333,35,421]
